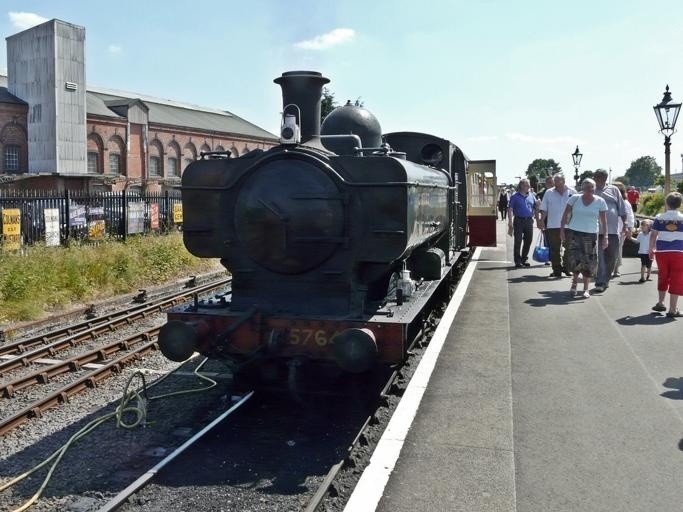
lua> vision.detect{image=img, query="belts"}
[517,217,532,219]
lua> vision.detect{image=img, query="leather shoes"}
[516,262,530,267]
[550,267,572,277]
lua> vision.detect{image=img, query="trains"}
[157,71,498,409]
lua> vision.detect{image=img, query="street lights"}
[653,85,682,212]
[572,145,582,188]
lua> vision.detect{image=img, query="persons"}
[496,169,681,318]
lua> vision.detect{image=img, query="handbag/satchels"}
[533,231,549,262]
[566,211,572,224]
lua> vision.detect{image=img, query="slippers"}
[667,308,683,316]
[652,303,666,311]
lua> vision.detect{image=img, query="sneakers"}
[596,282,609,291]
[639,278,652,282]
[614,272,620,277]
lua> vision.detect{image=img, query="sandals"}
[583,290,589,298]
[570,283,577,295]
